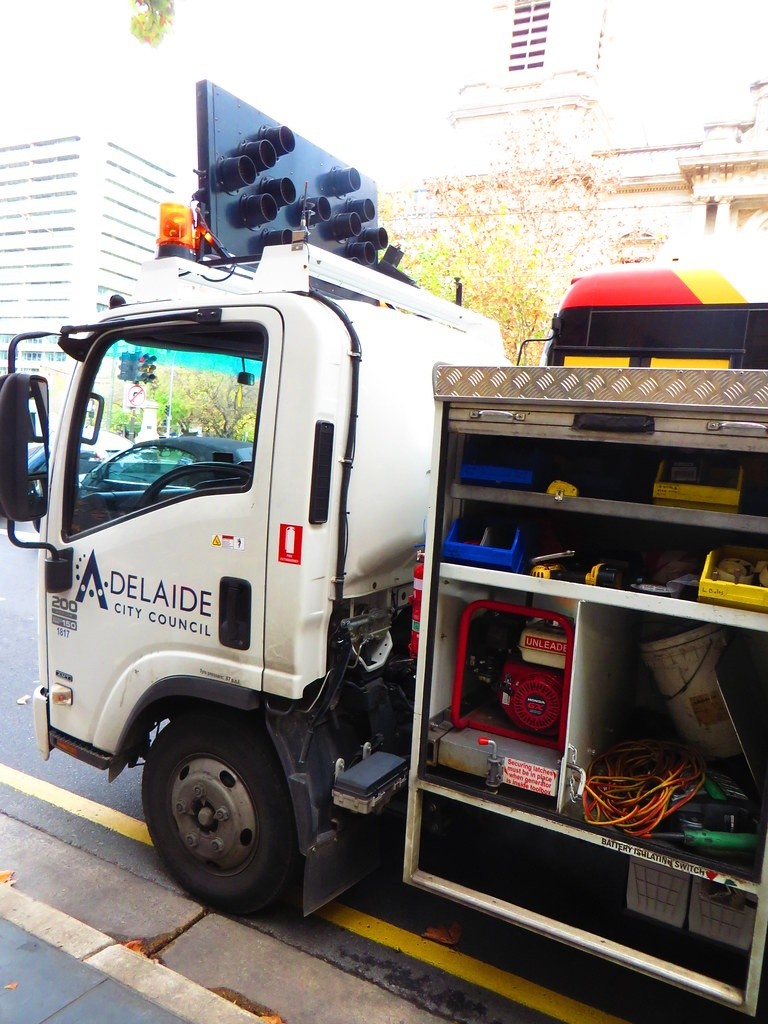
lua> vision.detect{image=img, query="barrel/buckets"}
[636,621,741,760]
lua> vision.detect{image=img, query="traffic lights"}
[116,350,157,385]
[164,404,170,417]
[163,419,167,427]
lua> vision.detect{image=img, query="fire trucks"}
[0,80,768,1024]
